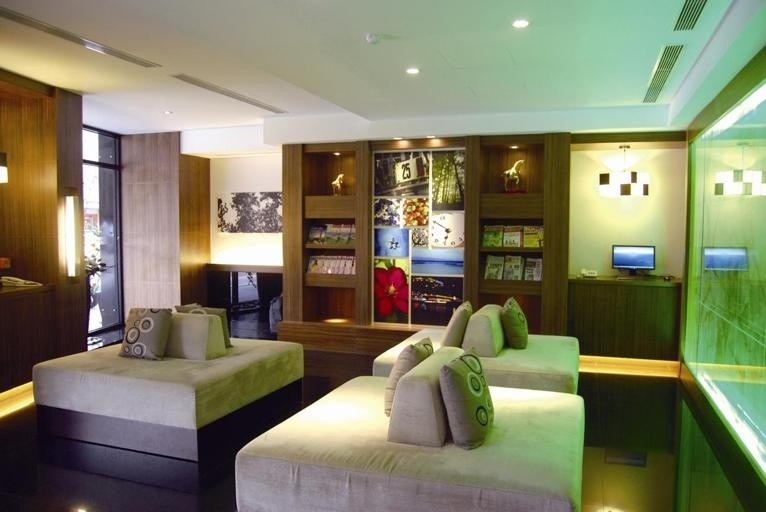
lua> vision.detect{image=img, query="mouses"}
[664,275,672,282]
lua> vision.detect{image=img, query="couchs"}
[371,324,580,395]
[32,337,303,496]
[233,375,587,512]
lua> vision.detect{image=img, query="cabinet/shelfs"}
[282,132,574,326]
[569,272,681,362]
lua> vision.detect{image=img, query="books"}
[523,258,543,281]
[524,226,543,248]
[307,223,355,246]
[305,256,355,274]
[503,225,523,247]
[483,225,504,247]
[483,255,505,279]
[502,256,524,280]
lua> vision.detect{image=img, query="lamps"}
[61,192,78,280]
[594,145,651,202]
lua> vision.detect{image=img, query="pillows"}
[501,294,529,349]
[116,306,174,363]
[174,303,200,312]
[176,307,234,349]
[383,337,434,417]
[438,298,472,352]
[438,351,494,451]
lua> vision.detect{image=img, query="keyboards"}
[616,274,647,280]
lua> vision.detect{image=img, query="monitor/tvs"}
[611,244,656,277]
[705,246,748,283]
